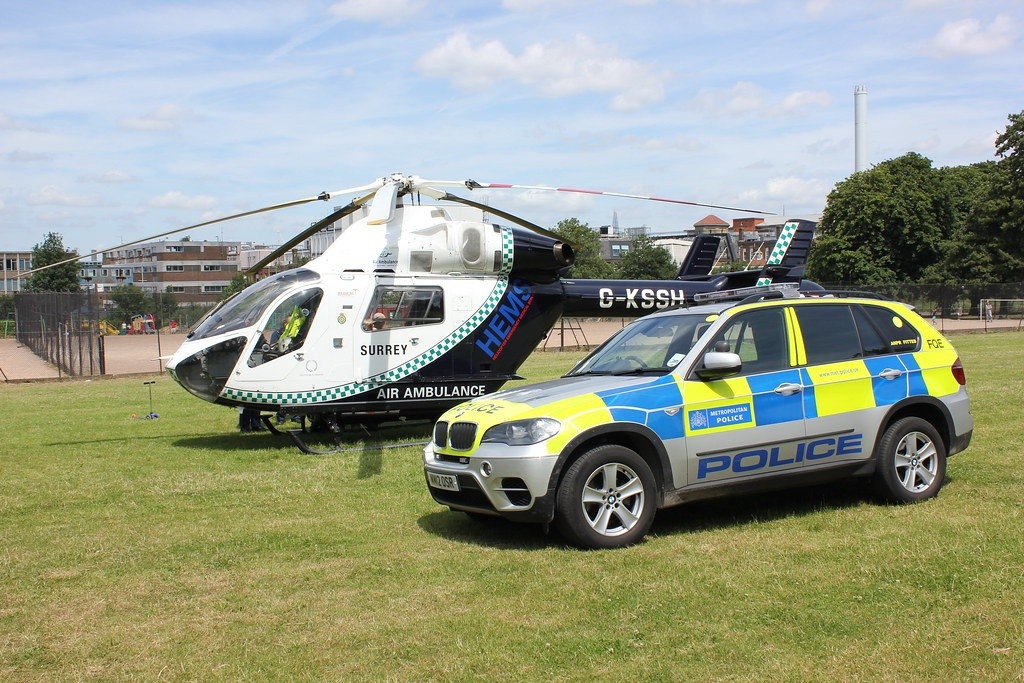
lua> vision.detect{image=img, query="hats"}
[372,313,386,322]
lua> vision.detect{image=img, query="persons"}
[956,304,963,320]
[270,314,306,425]
[170,320,177,334]
[372,313,386,329]
[985,302,992,322]
[121,320,132,335]
[932,308,937,325]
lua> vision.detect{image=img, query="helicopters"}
[11,175,820,458]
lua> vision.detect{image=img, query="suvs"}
[420,277,974,550]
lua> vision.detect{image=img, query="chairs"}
[698,325,724,348]
